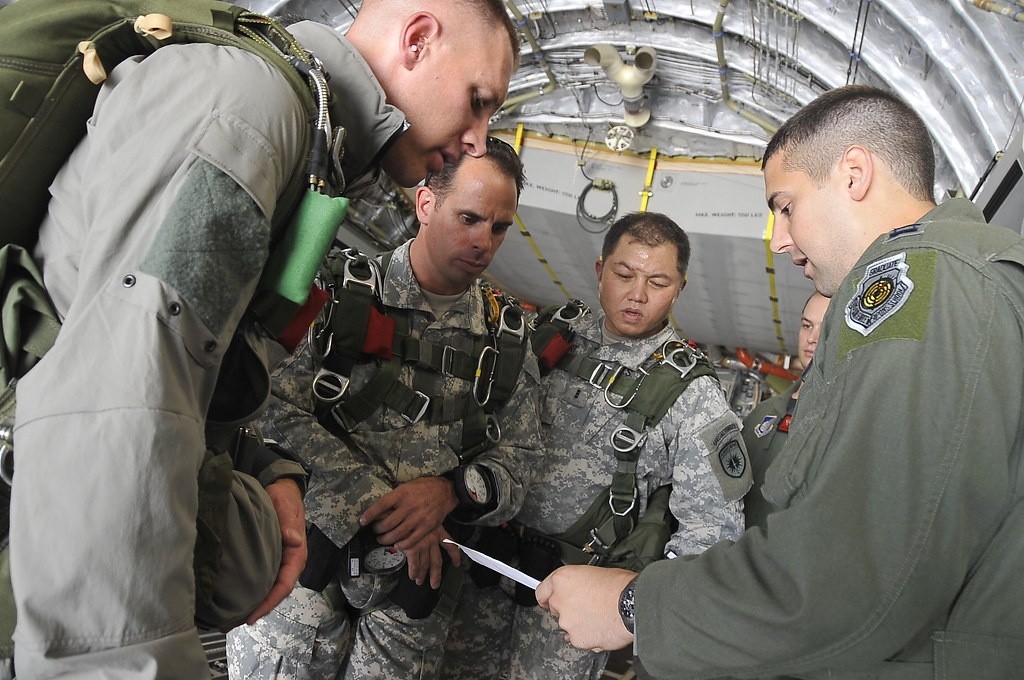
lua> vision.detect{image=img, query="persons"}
[740,288,832,519]
[1,0,533,680]
[222,134,547,680]
[436,207,754,680]
[530,83,1024,680]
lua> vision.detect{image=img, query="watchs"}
[617,571,637,634]
